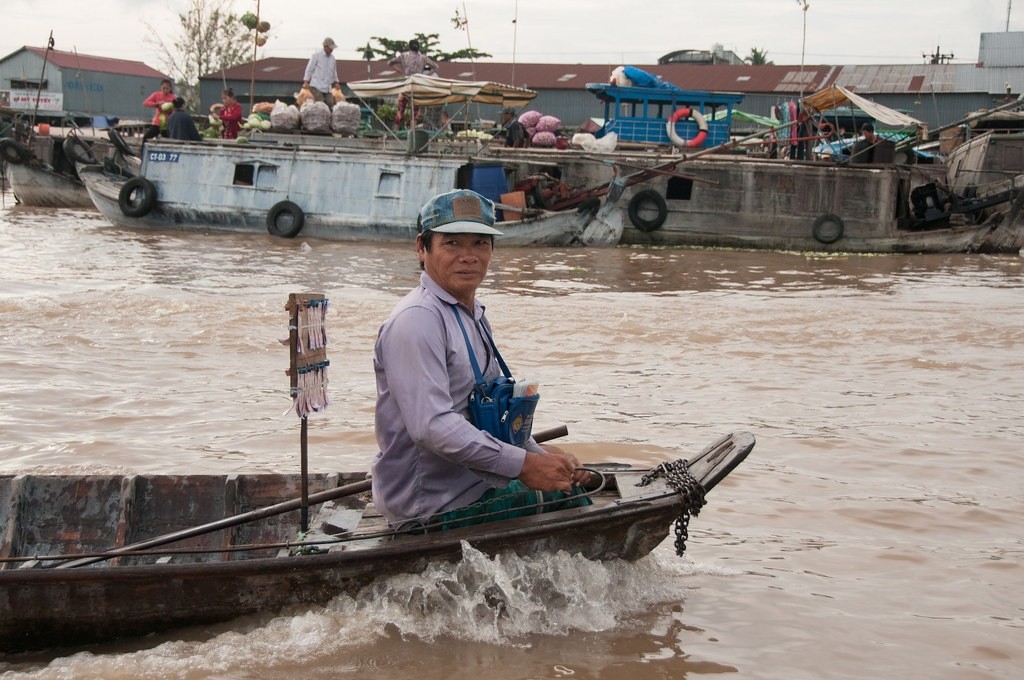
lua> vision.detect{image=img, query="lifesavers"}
[64,135,99,165]
[811,213,844,244]
[107,127,137,156]
[629,189,669,232]
[266,200,304,239]
[666,107,708,147]
[118,176,157,217]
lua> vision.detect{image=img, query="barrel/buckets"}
[850,140,871,163]
[872,140,896,164]
[38,122,50,136]
[471,162,508,222]
[500,191,527,220]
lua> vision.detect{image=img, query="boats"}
[0,106,1024,256]
[0,423,759,654]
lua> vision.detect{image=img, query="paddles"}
[59,425,570,571]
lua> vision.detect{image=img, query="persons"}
[858,124,884,146]
[303,38,340,113]
[441,111,451,131]
[168,97,203,145]
[388,40,439,131]
[140,78,177,161]
[497,107,524,148]
[372,188,591,535]
[219,87,243,140]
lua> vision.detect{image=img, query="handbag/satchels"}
[332,87,346,105]
[295,88,315,106]
[449,304,539,444]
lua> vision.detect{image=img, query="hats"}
[498,106,516,114]
[322,37,337,49]
[417,189,503,237]
